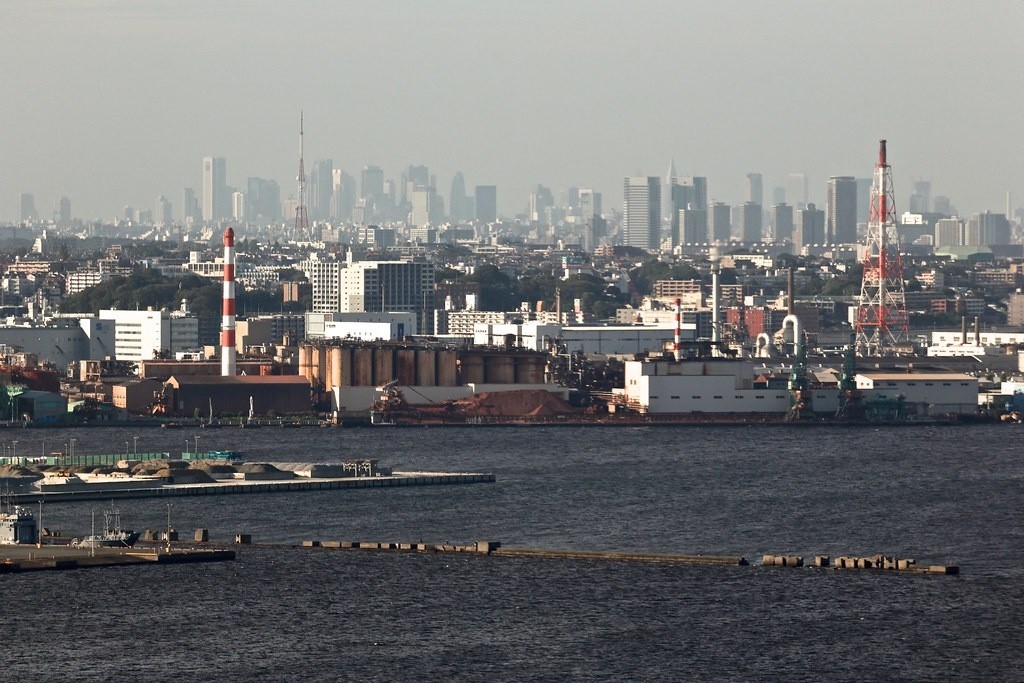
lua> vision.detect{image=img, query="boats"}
[279,421,301,428]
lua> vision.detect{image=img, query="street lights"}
[133,437,138,460]
[70,439,76,467]
[91,508,96,557]
[38,500,44,545]
[12,440,18,456]
[186,440,188,452]
[166,503,174,546]
[126,442,128,461]
[64,444,67,466]
[195,436,200,453]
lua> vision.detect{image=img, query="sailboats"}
[242,396,262,429]
[202,397,223,429]
